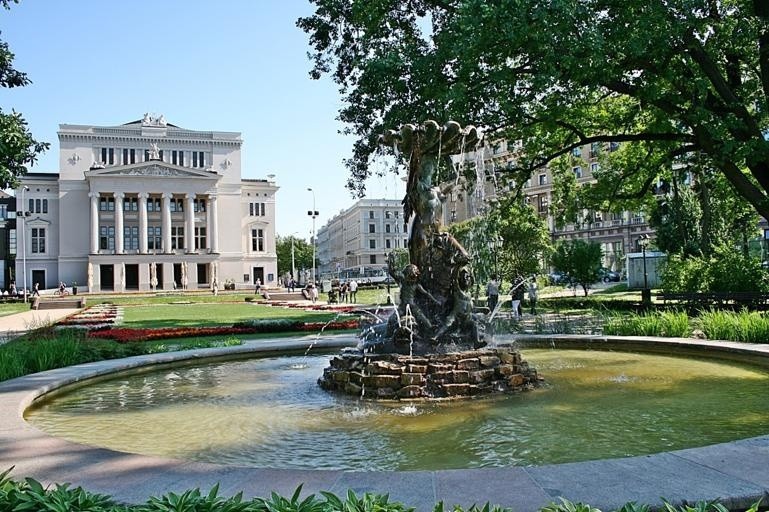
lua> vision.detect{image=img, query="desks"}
[487,235,505,281]
[306,188,316,289]
[388,211,398,248]
[637,232,650,290]
[292,231,299,282]
[17,184,31,305]
[450,208,457,224]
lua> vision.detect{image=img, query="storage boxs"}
[0,293,31,303]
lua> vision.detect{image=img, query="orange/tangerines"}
[327,291,338,305]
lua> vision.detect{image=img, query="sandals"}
[316,264,394,286]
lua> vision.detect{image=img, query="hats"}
[599,267,621,283]
[547,270,566,282]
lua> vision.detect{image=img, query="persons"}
[255,278,262,294]
[331,275,358,304]
[33,283,39,296]
[59,281,65,294]
[213,278,218,295]
[528,277,538,315]
[485,274,499,315]
[288,276,293,292]
[291,277,296,293]
[510,276,524,321]
[308,285,319,303]
